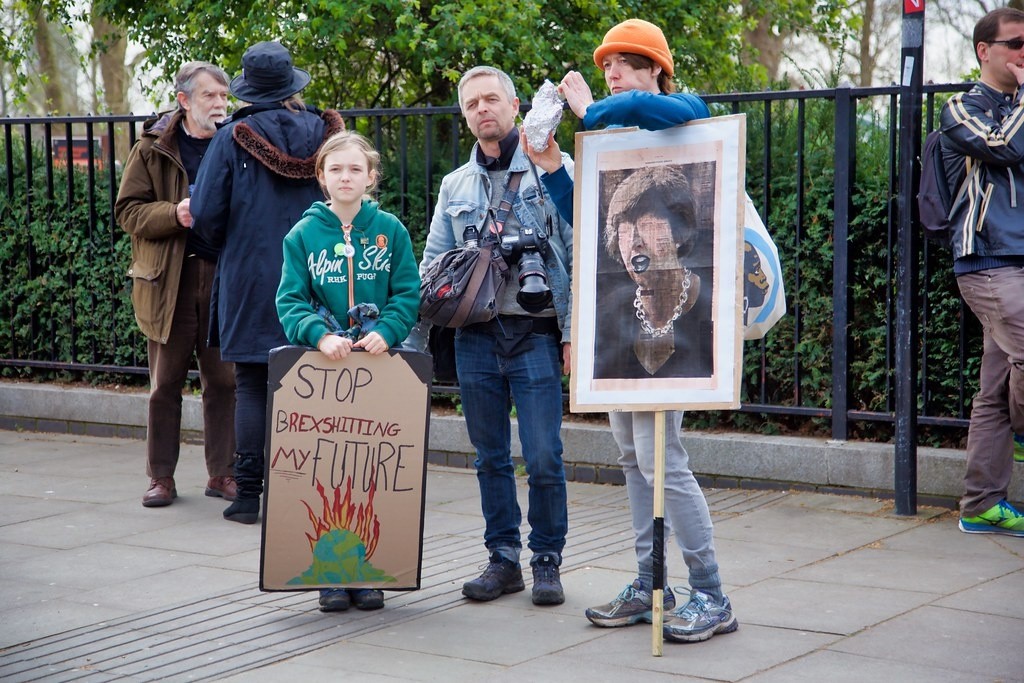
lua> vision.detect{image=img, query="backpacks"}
[916,95,1001,232]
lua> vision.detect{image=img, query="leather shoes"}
[142,477,176,506]
[204,476,238,501]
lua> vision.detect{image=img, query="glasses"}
[985,40,1024,49]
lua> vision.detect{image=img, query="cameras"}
[501,226,554,314]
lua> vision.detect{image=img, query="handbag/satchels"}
[418,246,503,328]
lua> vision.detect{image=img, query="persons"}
[115,63,238,506]
[190,45,345,523]
[418,65,574,605]
[521,19,738,642]
[939,7,1024,537]
[275,134,422,608]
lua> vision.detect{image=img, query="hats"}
[229,41,311,104]
[593,18,674,78]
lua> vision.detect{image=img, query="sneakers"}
[585,579,676,627]
[349,589,385,609]
[318,589,350,611]
[958,499,1024,536]
[663,586,737,641]
[1012,432,1024,461]
[532,554,565,605]
[462,550,524,601]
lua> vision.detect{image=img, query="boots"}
[223,454,264,524]
